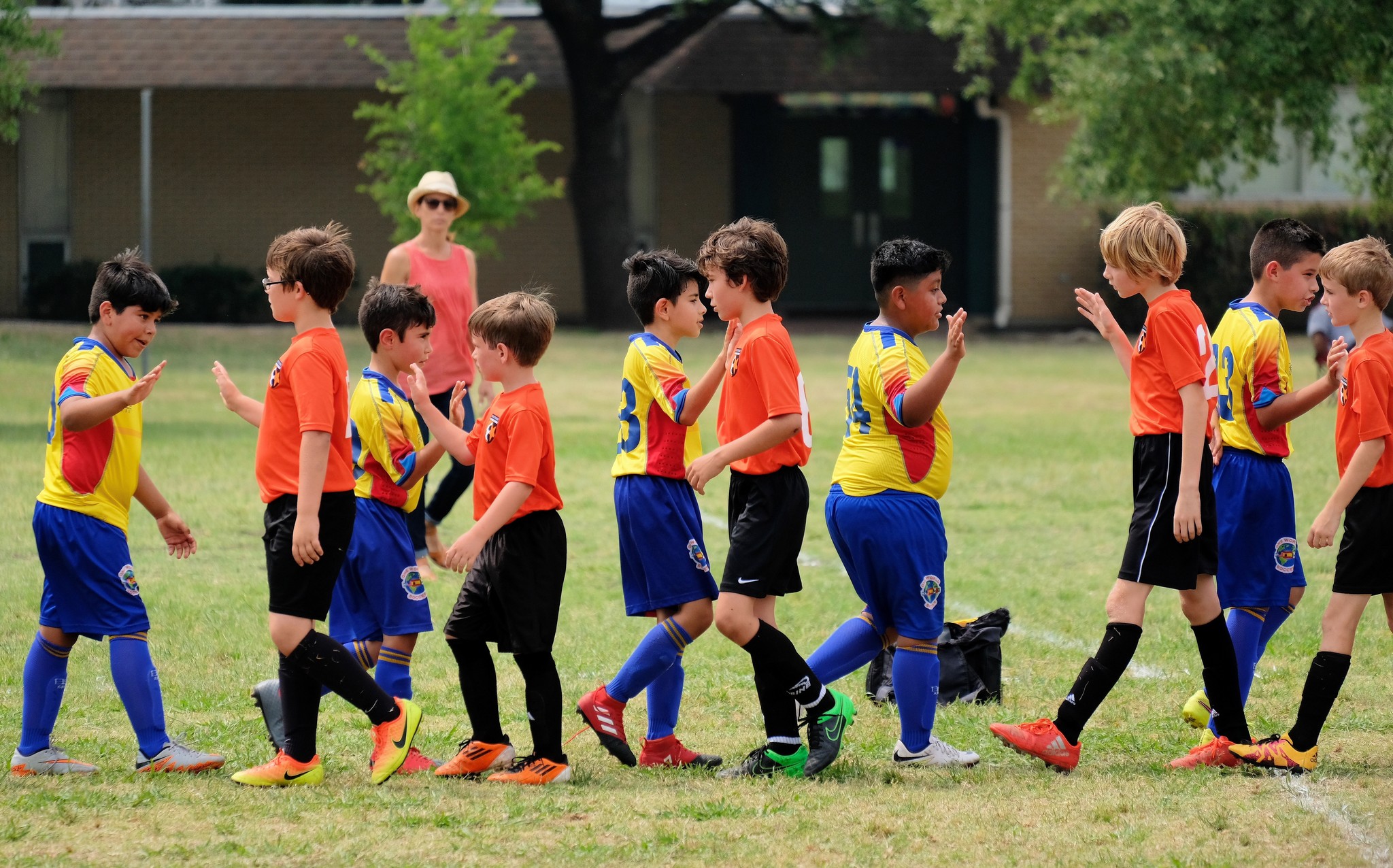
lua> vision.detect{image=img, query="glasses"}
[262,277,310,296]
[424,196,457,211]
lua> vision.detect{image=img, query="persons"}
[1307,289,1392,370]
[1182,216,1347,752]
[251,283,442,775]
[378,171,479,581]
[211,224,422,790]
[989,204,1255,774]
[1227,235,1393,774]
[11,248,226,777]
[686,219,858,780]
[576,250,739,769]
[408,288,573,787]
[763,239,981,766]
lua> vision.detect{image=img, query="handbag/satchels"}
[866,608,1011,706]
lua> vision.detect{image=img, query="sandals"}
[422,521,450,570]
[411,558,430,570]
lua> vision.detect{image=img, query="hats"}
[407,170,470,221]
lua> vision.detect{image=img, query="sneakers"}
[488,751,572,785]
[1182,691,1212,730]
[575,681,637,768]
[891,735,979,770]
[250,680,287,755]
[136,732,226,773]
[10,742,98,777]
[369,695,422,786]
[1199,730,1257,747]
[434,734,515,777]
[716,740,809,781]
[990,718,1083,776]
[804,687,857,775]
[1164,736,1243,770]
[1228,731,1318,774]
[370,745,448,776]
[638,732,723,771]
[230,749,324,786]
